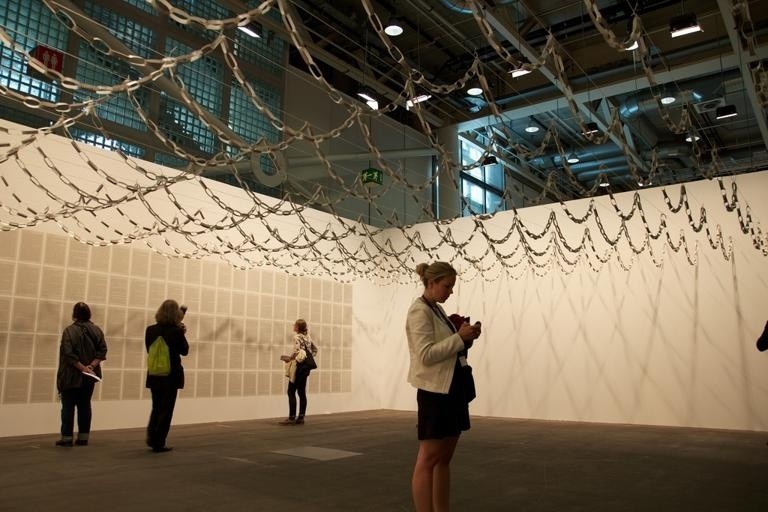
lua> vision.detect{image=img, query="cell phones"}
[474,321,481,324]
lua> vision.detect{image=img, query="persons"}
[405,261,481,511]
[277,318,319,424]
[144,298,189,453]
[55,301,107,447]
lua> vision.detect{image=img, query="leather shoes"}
[153,445,169,452]
[75,439,88,445]
[56,440,73,447]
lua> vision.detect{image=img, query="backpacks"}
[148,336,171,376]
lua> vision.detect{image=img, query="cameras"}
[180,306,187,313]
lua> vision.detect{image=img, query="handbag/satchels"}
[296,343,317,370]
